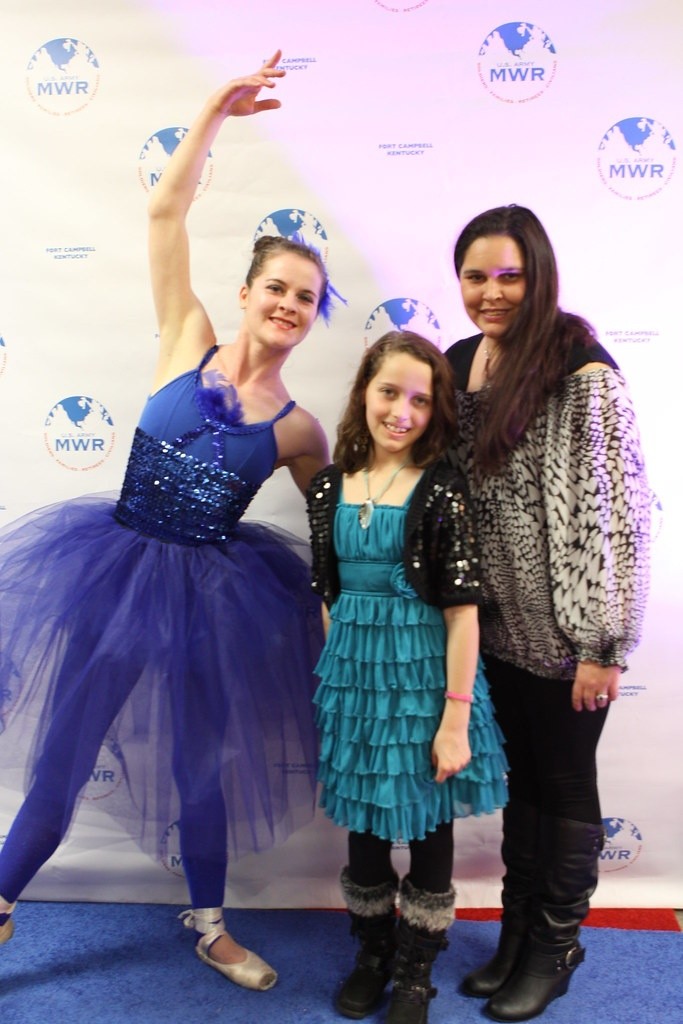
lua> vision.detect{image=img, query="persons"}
[0,49,332,989]
[306,331,481,1024]
[440,203,650,1024]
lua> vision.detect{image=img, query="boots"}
[336,865,400,1019]
[458,807,543,998]
[382,874,456,1024]
[484,818,605,1023]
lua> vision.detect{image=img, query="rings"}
[596,693,608,701]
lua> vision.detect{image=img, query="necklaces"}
[357,457,412,529]
[482,336,494,390]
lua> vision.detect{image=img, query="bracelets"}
[445,691,472,702]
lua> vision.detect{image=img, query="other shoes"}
[177,909,278,991]
